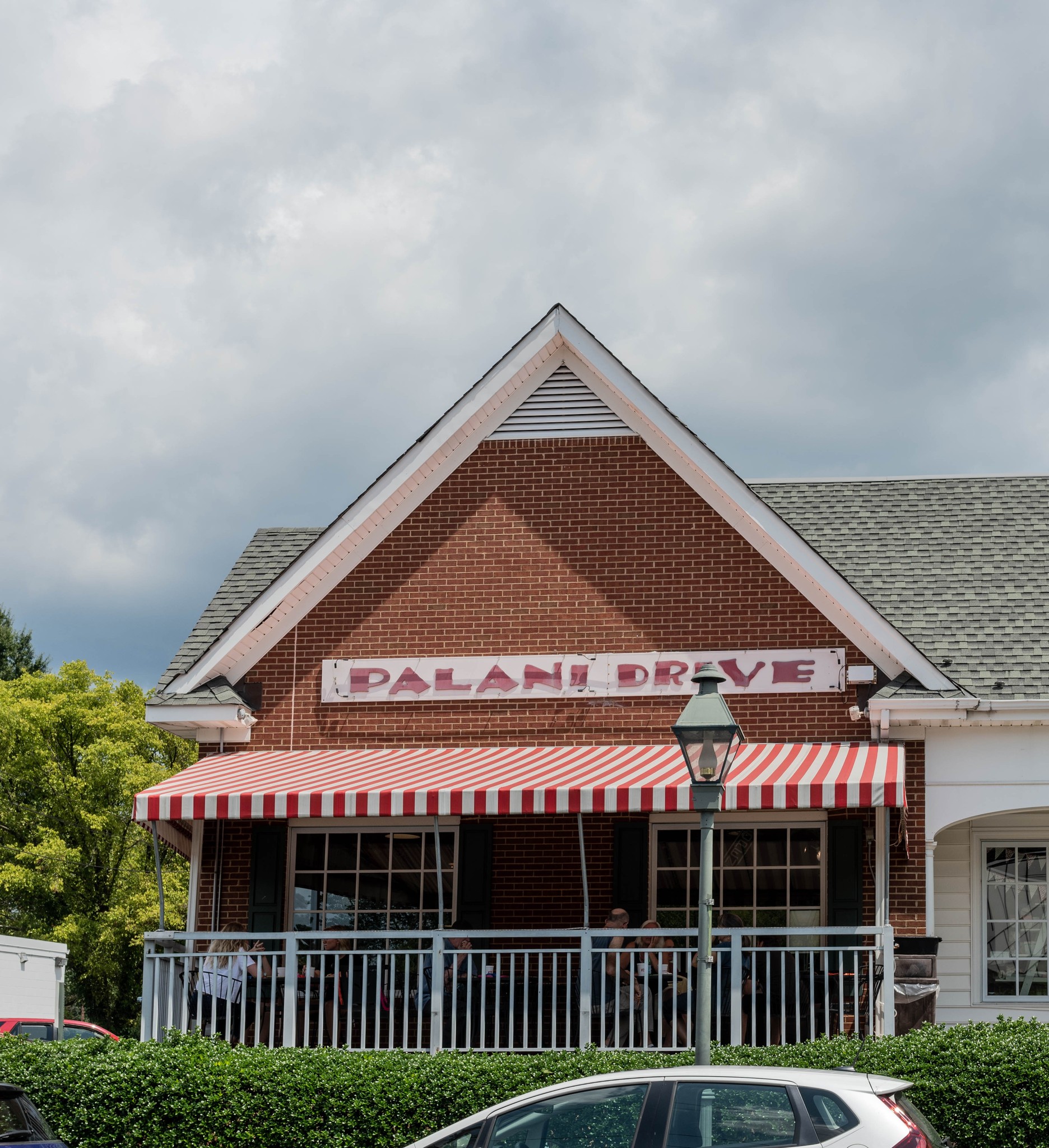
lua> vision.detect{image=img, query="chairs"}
[561,964,886,1047]
[179,966,554,1055]
[670,1111,703,1148]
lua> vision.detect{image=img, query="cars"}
[402,1062,955,1148]
[0,1017,121,1044]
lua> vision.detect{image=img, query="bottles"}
[648,953,652,976]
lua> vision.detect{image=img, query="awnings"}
[130,740,908,864]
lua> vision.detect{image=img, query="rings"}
[254,945,257,948]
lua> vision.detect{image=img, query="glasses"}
[322,939,327,941]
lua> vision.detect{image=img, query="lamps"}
[237,709,258,729]
[54,957,69,967]
[20,954,29,963]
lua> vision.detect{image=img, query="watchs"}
[326,973,332,977]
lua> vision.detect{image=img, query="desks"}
[448,974,545,1046]
[246,973,346,1046]
[628,971,686,1043]
[805,970,880,1033]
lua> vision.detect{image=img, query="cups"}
[486,966,494,975]
[305,967,315,978]
[277,967,285,977]
[637,963,644,976]
[657,964,669,974]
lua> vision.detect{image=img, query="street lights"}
[667,660,748,1148]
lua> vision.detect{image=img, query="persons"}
[662,912,752,1047]
[750,935,813,1048]
[195,921,272,1046]
[413,920,480,1049]
[576,908,657,1049]
[302,925,377,1049]
[620,920,670,979]
